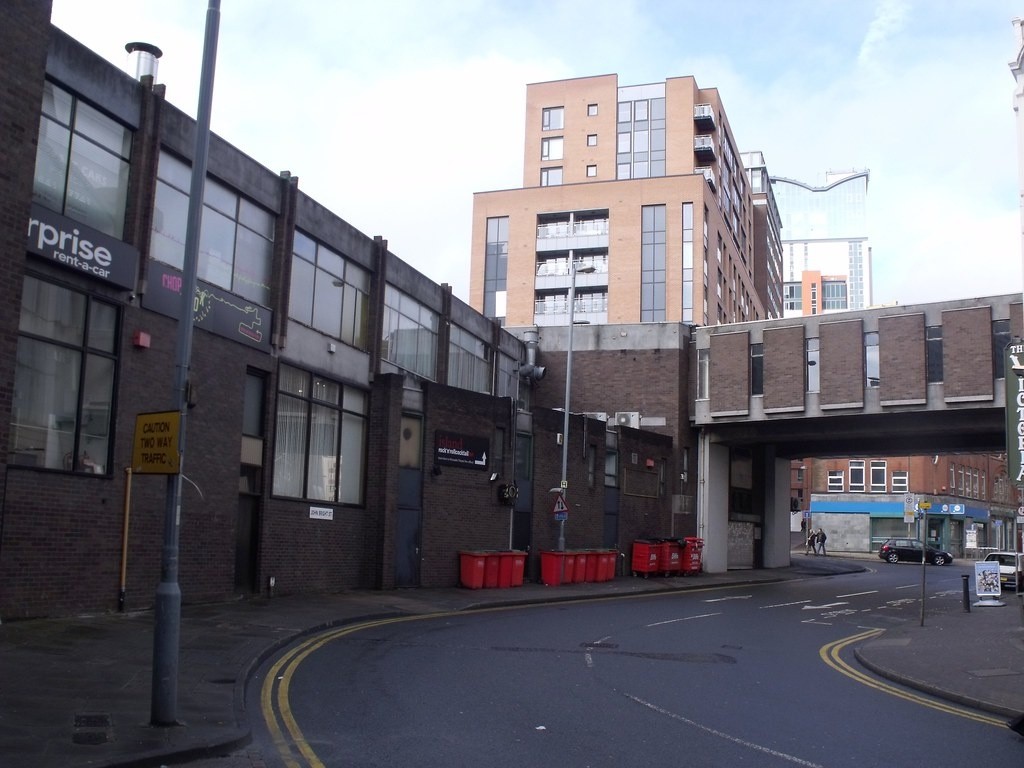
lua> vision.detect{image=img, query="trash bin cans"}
[498,548,514,589]
[540,548,619,584]
[460,550,488,590]
[679,536,704,578]
[659,535,684,577]
[631,537,663,579]
[511,548,529,587]
[483,549,500,588]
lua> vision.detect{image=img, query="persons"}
[805,529,817,555]
[801,519,806,532]
[815,528,827,557]
[792,498,799,515]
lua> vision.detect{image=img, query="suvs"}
[879,539,953,565]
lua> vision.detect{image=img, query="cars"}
[983,551,1024,591]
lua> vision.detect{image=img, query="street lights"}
[556,267,596,551]
